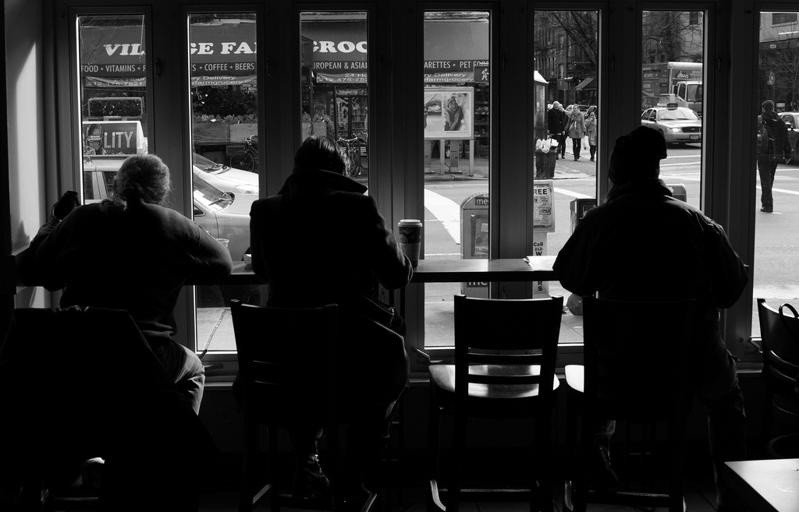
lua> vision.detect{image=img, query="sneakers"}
[760,207,773,213]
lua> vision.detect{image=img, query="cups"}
[398,218,422,271]
[243,254,252,270]
[215,238,229,249]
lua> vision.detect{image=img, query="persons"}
[757,99,794,214]
[444,93,470,130]
[549,99,598,162]
[250,134,414,512]
[553,126,749,511]
[20,152,231,511]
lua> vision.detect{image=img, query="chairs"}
[232,296,400,509]
[12,308,174,511]
[756,296,798,462]
[563,295,702,511]
[427,294,563,511]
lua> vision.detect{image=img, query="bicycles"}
[353,133,368,177]
[230,134,259,172]
[337,135,362,177]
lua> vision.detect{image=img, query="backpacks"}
[756,115,773,163]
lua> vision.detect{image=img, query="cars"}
[757,113,799,165]
[584,106,597,119]
[642,94,702,144]
[564,105,589,120]
[81,121,259,199]
[84,159,259,270]
[547,103,564,112]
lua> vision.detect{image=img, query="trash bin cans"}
[535,149,555,178]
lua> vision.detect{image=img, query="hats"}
[605,123,671,187]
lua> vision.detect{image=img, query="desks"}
[188,259,585,379]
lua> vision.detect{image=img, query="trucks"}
[642,62,703,121]
